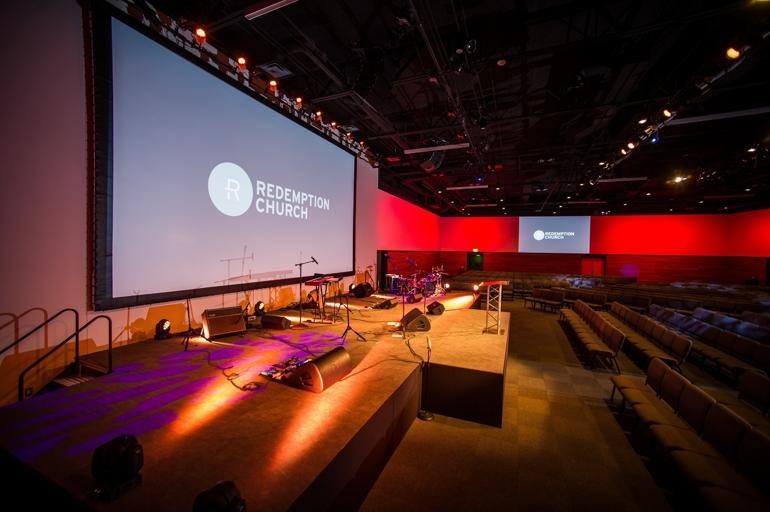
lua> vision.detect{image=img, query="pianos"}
[306,274,343,286]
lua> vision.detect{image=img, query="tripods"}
[342,300,366,349]
[173,298,202,351]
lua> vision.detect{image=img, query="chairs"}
[558,298,769,509]
[444,270,769,312]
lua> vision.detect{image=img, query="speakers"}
[203,303,247,339]
[427,300,446,315]
[353,280,374,296]
[399,307,431,332]
[406,293,423,304]
[287,346,354,393]
[261,314,291,330]
[380,297,398,309]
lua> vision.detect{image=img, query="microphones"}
[311,255,317,264]
